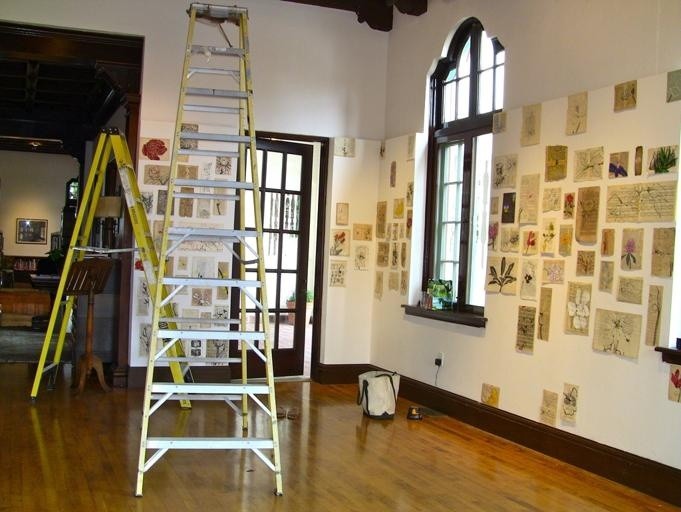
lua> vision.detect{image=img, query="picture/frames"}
[16,218,47,245]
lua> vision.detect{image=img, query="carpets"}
[0,327,75,365]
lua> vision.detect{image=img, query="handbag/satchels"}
[356,370,402,421]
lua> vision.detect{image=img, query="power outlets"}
[433,352,443,367]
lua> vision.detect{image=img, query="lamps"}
[93,196,122,257]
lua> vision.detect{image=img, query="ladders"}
[29,125,196,411]
[135,1,283,497]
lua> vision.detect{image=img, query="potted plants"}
[287,290,314,327]
